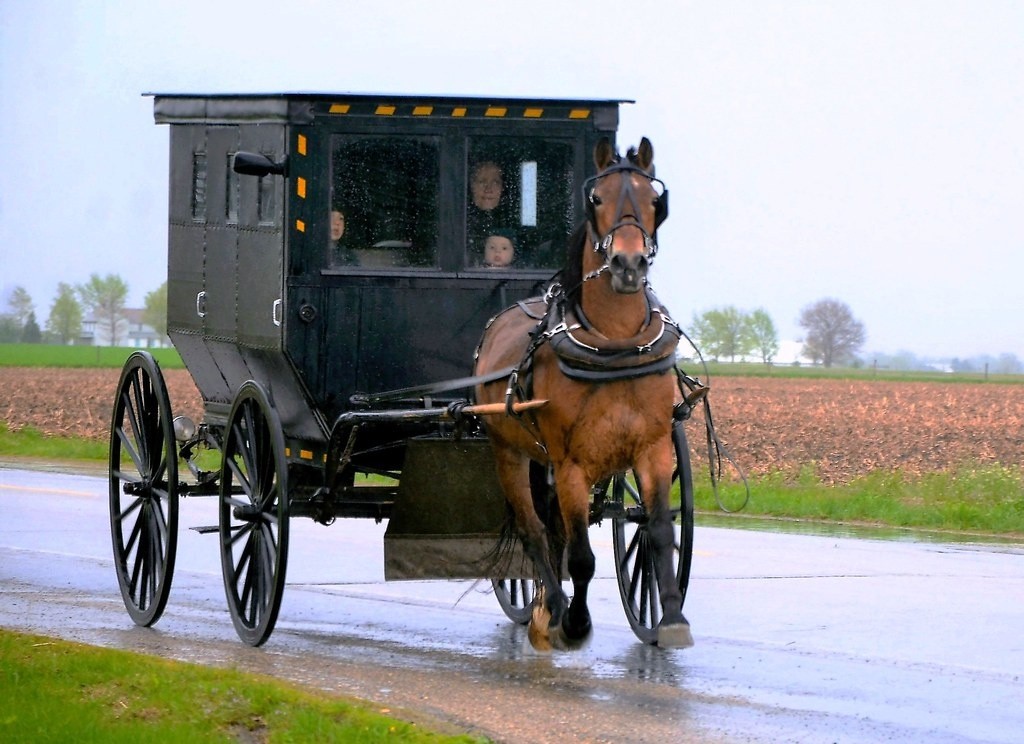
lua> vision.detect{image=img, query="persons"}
[331,195,361,268]
[482,229,519,269]
[415,161,570,269]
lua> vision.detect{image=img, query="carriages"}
[108,91,710,651]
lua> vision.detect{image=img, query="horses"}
[465,134,697,661]
